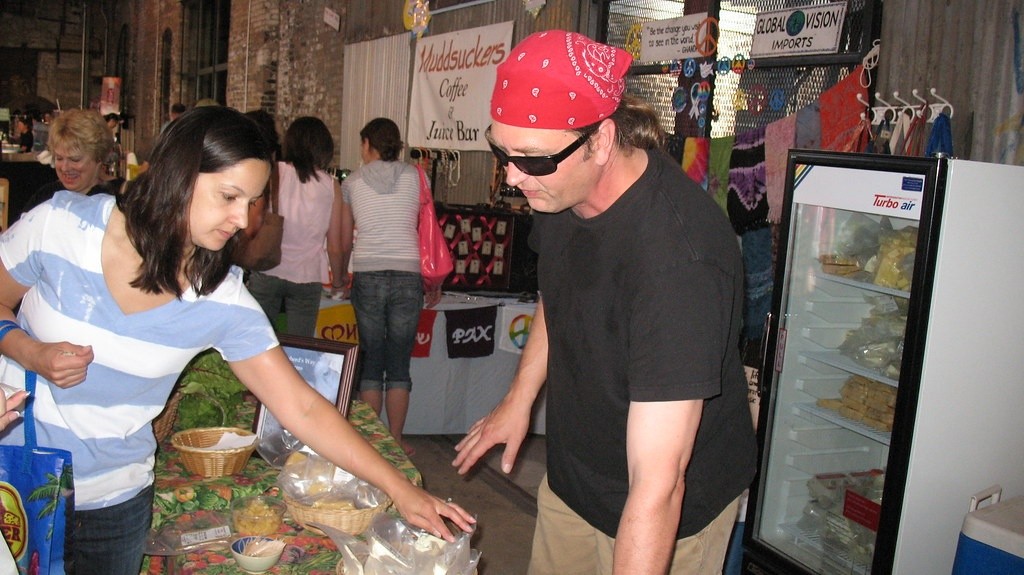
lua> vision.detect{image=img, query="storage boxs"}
[950,486,1024,575]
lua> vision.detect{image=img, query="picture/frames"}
[250,332,359,461]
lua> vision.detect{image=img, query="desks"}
[137,389,423,575]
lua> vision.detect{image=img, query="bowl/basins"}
[230,535,283,574]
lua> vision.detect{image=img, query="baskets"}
[151,392,181,443]
[169,426,260,477]
[283,490,392,537]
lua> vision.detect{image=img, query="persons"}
[1,119,33,153]
[246,116,348,339]
[340,117,441,457]
[160,103,185,135]
[103,113,118,128]
[0,99,480,575]
[447,28,757,575]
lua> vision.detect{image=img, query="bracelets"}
[332,281,344,288]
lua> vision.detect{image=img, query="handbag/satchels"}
[416,163,452,289]
[0,319,74,575]
[236,163,283,272]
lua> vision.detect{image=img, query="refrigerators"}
[731,145,1023,575]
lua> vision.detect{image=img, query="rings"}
[444,497,452,504]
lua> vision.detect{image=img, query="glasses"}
[484,124,598,177]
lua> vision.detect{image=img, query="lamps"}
[257,44,274,68]
